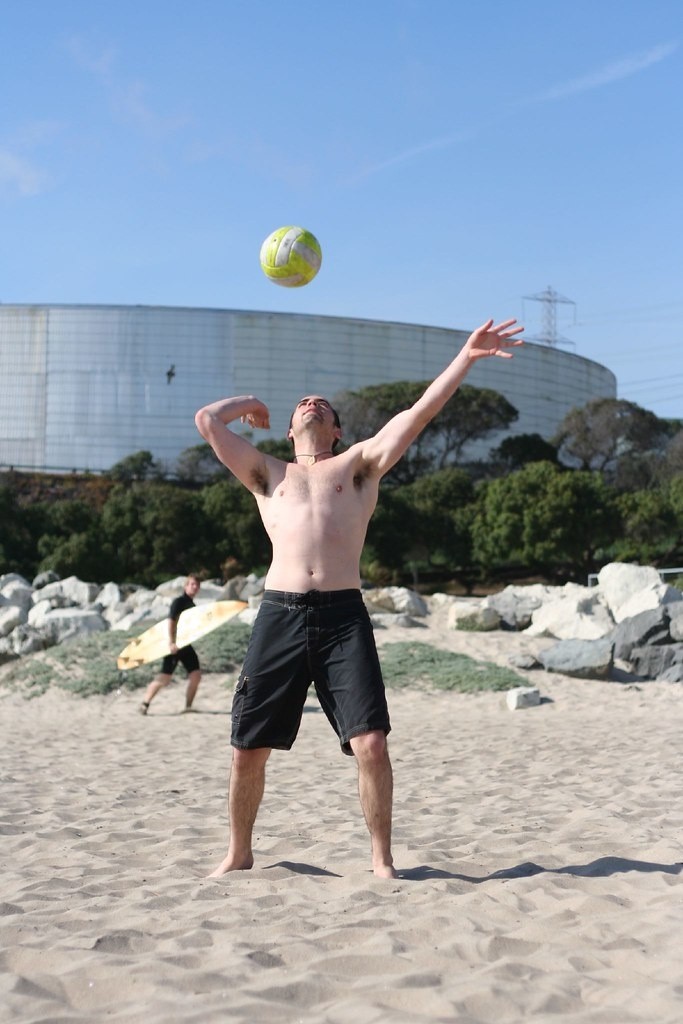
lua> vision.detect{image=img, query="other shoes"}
[138,702,148,714]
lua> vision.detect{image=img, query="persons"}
[137,576,202,716]
[194,317,525,877]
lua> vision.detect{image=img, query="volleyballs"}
[258,223,322,288]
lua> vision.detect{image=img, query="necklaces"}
[292,451,336,465]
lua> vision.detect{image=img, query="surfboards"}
[115,598,248,671]
